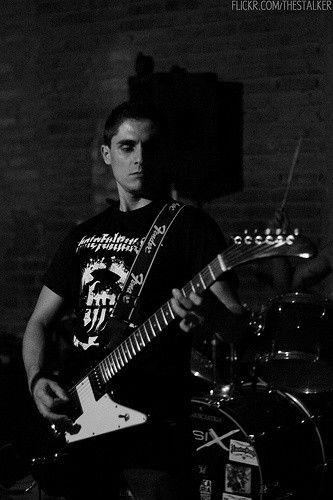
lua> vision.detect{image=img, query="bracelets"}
[29,372,48,397]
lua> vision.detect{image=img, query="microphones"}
[211,334,222,385]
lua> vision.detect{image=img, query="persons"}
[267,207,333,295]
[23,100,247,500]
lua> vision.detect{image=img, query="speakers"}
[126,70,224,202]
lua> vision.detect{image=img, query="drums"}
[192,330,238,384]
[253,292,333,394]
[189,384,333,500]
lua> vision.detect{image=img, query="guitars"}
[16,224,313,500]
